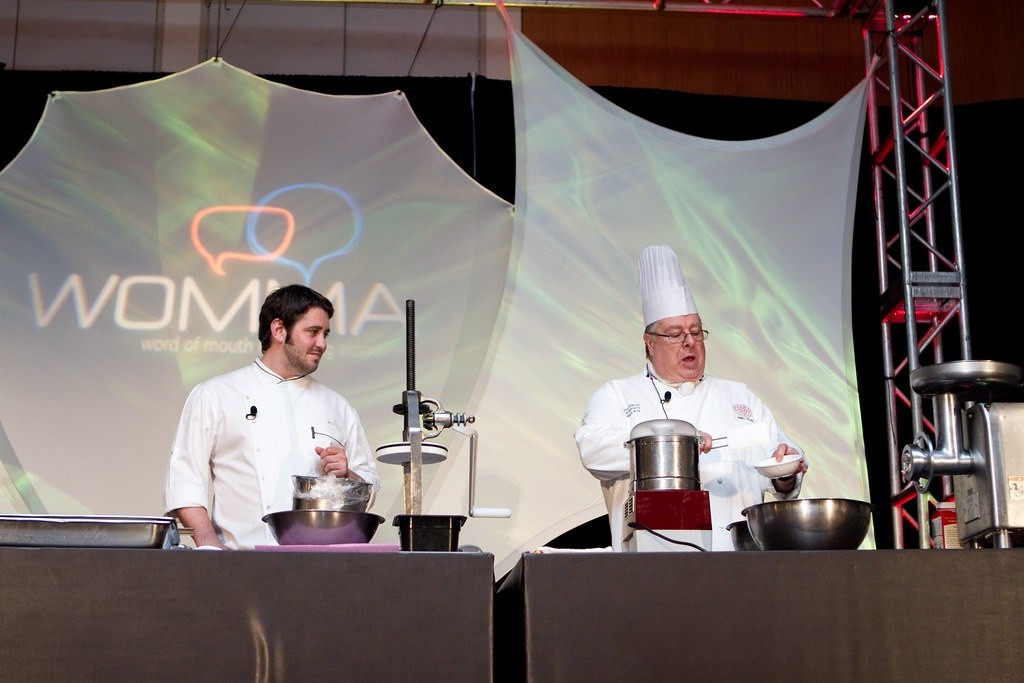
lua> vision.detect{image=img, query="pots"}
[623,419,704,491]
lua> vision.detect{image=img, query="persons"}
[574,288,809,552]
[165,284,378,550]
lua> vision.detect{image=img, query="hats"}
[637,245,699,328]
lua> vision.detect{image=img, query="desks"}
[497,548,1023,683]
[0,548,493,682]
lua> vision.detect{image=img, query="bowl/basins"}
[725,498,877,551]
[753,454,802,479]
[290,474,372,513]
[261,510,385,544]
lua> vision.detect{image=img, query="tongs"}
[701,437,729,450]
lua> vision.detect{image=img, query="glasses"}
[644,330,709,343]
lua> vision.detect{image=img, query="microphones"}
[664,391,672,401]
[250,406,257,416]
